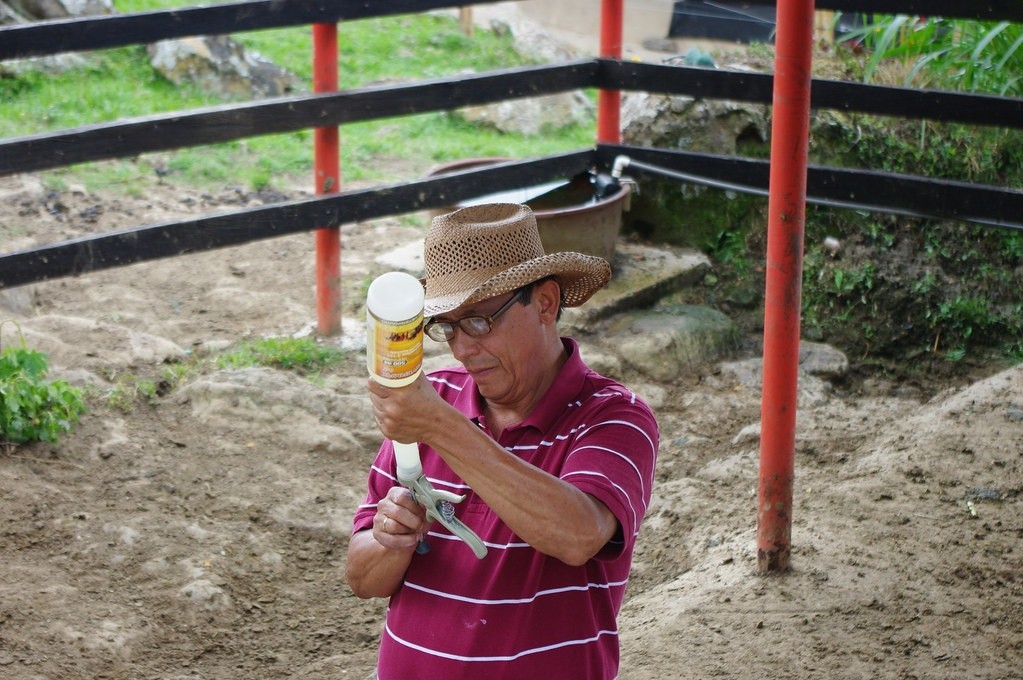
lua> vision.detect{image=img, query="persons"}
[346,207,657,679]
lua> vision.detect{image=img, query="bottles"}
[366,271,425,390]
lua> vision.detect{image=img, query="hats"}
[417,202,614,309]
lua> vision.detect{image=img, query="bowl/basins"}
[421,159,631,280]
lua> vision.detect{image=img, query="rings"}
[383,516,389,532]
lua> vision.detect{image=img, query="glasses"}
[424,290,525,343]
